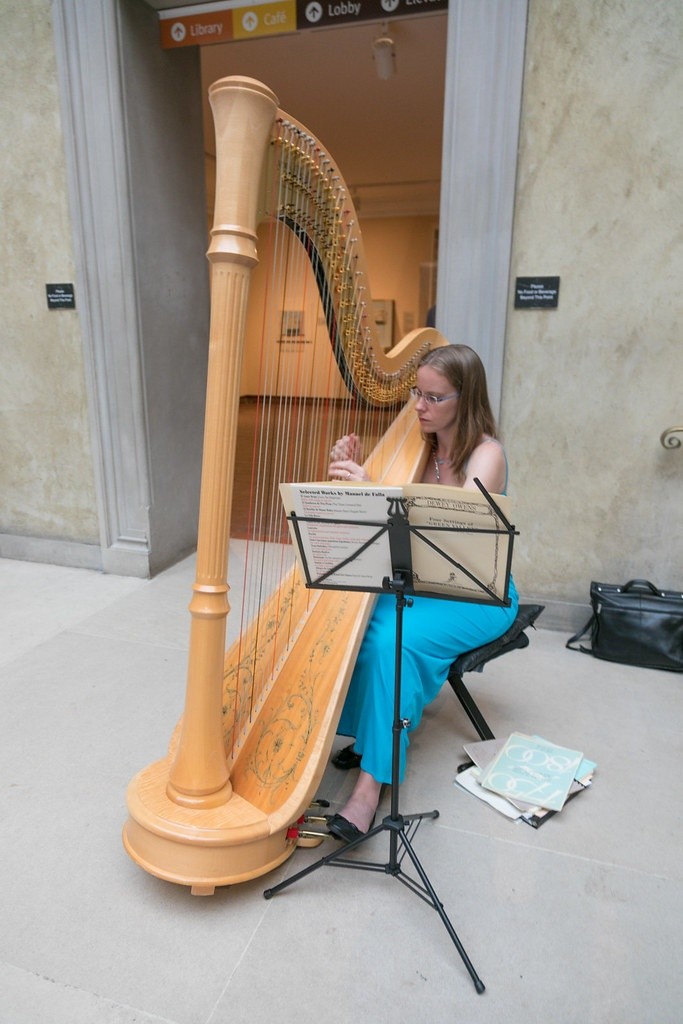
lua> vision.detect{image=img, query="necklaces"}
[433,448,439,485]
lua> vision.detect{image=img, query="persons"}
[327,345,521,846]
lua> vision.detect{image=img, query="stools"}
[448,604,546,774]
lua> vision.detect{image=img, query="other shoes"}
[331,743,363,769]
[327,812,376,844]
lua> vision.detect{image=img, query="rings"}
[346,472,351,480]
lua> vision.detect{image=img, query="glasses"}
[408,385,459,406]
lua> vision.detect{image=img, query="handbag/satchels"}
[566,579,683,674]
